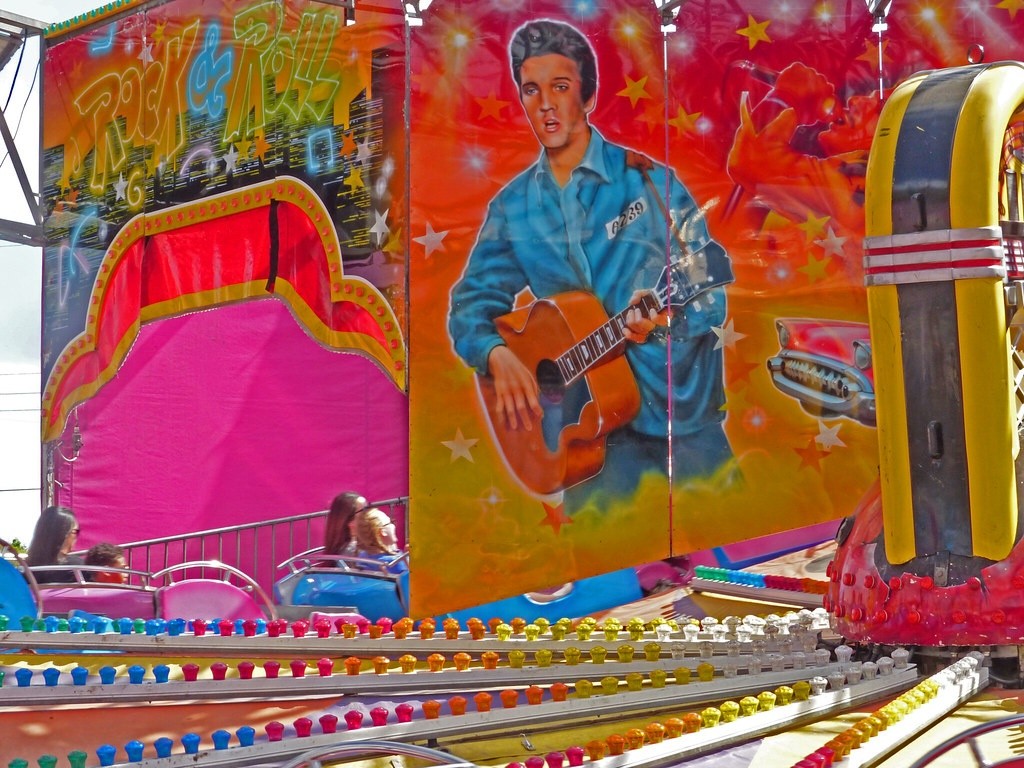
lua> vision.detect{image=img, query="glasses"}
[354,502,372,514]
[383,519,395,527]
[65,528,80,538]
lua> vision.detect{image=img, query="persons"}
[21,506,130,585]
[323,491,409,575]
[635,557,694,599]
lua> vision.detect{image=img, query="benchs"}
[0,538,409,631]
